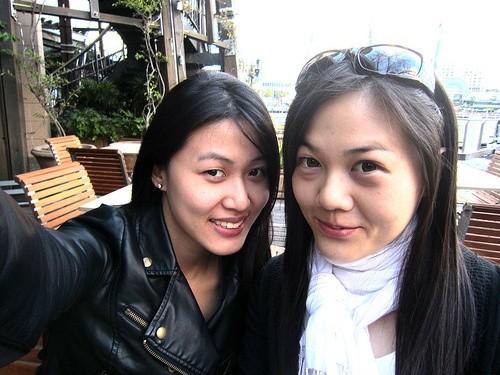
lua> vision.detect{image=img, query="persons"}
[0,71,281,375]
[234,43,500,375]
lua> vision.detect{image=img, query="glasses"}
[295,44,446,137]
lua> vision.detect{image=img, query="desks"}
[459,203,500,266]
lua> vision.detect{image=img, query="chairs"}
[13,135,132,231]
